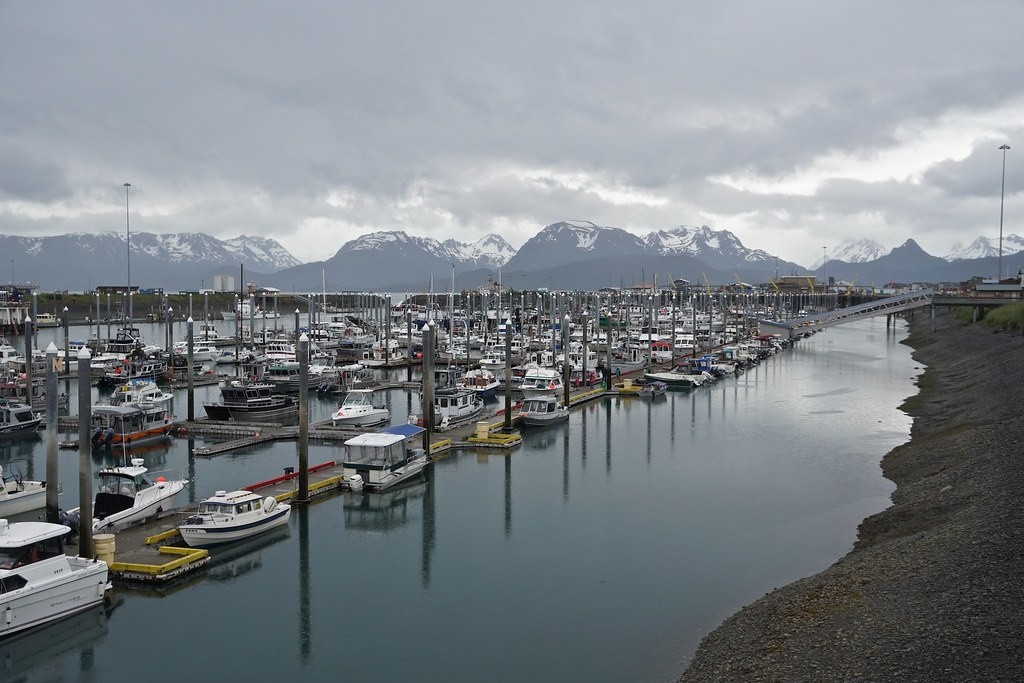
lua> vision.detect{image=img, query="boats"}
[0,284,831,640]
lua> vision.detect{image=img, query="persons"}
[589,375,595,388]
[615,367,622,383]
[602,367,607,382]
